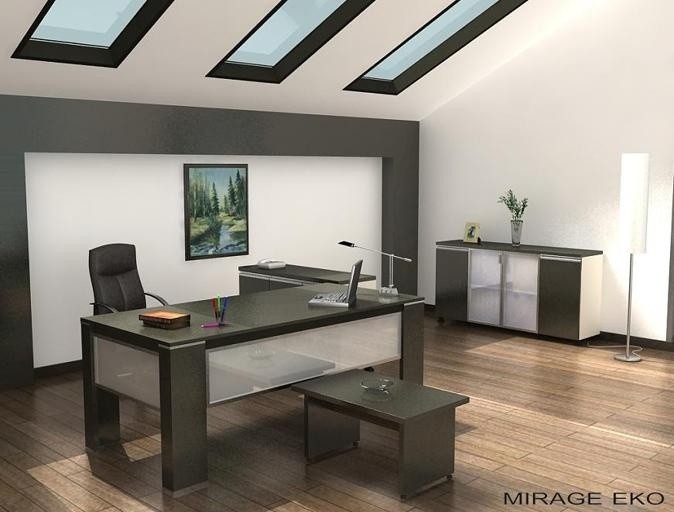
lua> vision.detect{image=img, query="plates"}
[360,378,394,392]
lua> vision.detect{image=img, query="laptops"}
[308,259,363,308]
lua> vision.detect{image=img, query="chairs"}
[89,244,170,314]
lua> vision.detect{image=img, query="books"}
[138,309,191,331]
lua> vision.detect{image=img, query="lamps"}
[338,240,413,297]
[586,152,651,363]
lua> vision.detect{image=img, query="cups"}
[509,219,523,247]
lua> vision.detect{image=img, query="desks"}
[79,282,426,499]
[290,369,471,499]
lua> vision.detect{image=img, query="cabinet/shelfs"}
[239,263,378,297]
[435,240,604,344]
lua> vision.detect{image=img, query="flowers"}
[497,188,529,220]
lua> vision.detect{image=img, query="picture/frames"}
[183,163,249,261]
[464,222,482,243]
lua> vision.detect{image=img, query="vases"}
[510,219,524,245]
